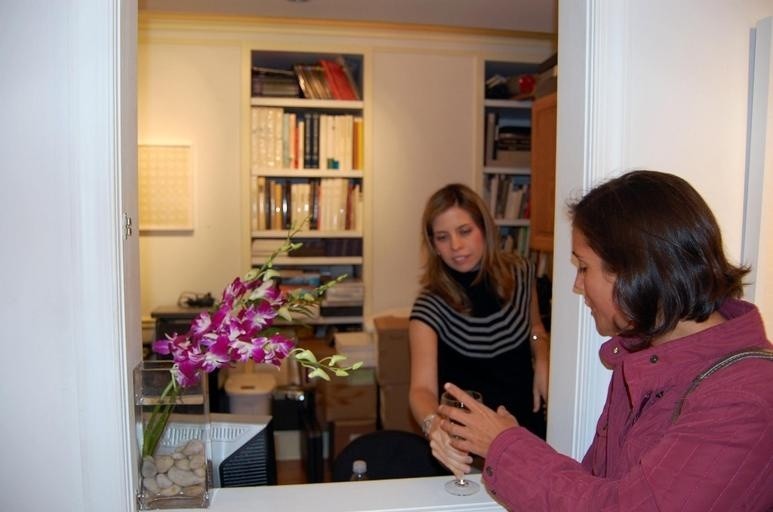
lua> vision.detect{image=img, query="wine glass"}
[440,389,481,496]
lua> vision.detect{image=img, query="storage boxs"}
[381,386,420,431]
[373,317,412,385]
[325,365,377,422]
[333,422,376,458]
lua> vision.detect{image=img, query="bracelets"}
[421,412,439,437]
[529,331,549,345]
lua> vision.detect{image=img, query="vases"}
[132,360,213,511]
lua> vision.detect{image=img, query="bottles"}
[349,458,370,482]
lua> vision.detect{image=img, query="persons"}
[407,182,551,482]
[434,168,773,511]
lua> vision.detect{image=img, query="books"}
[254,263,364,319]
[250,105,364,170]
[484,173,530,219]
[251,175,363,231]
[485,112,532,169]
[251,55,360,100]
[495,225,547,279]
[251,239,363,256]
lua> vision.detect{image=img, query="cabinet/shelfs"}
[473,50,557,278]
[240,45,373,333]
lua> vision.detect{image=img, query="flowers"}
[142,216,363,456]
